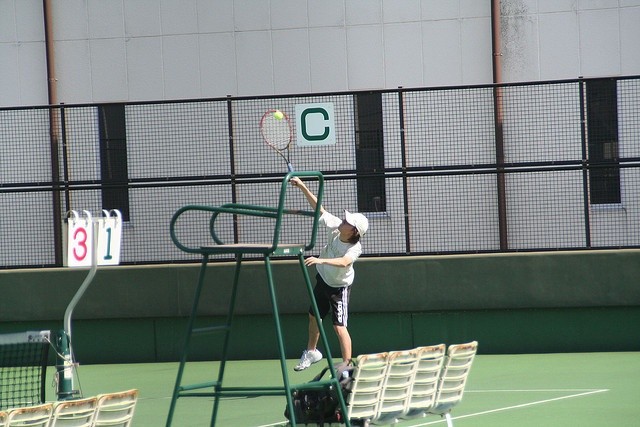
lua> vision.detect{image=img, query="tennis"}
[274,111,283,120]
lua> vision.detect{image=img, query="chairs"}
[6,402,53,427]
[164,169,355,426]
[405,343,447,421]
[0,410,9,427]
[91,388,139,426]
[51,395,98,426]
[347,352,388,427]
[425,341,479,427]
[374,347,421,427]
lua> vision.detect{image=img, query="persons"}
[289,174,369,378]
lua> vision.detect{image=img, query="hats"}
[344,209,369,237]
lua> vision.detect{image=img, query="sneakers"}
[329,362,354,377]
[294,349,323,371]
